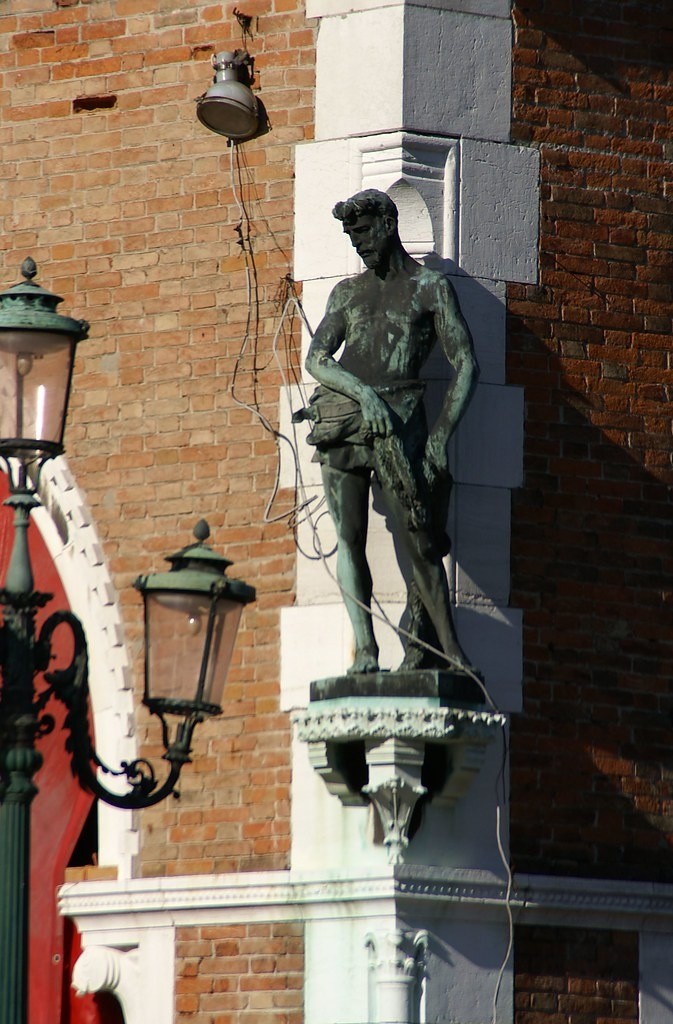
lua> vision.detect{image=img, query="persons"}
[290,185,484,681]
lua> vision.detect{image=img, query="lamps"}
[193,47,263,141]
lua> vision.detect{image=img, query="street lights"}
[1,252,255,1021]
